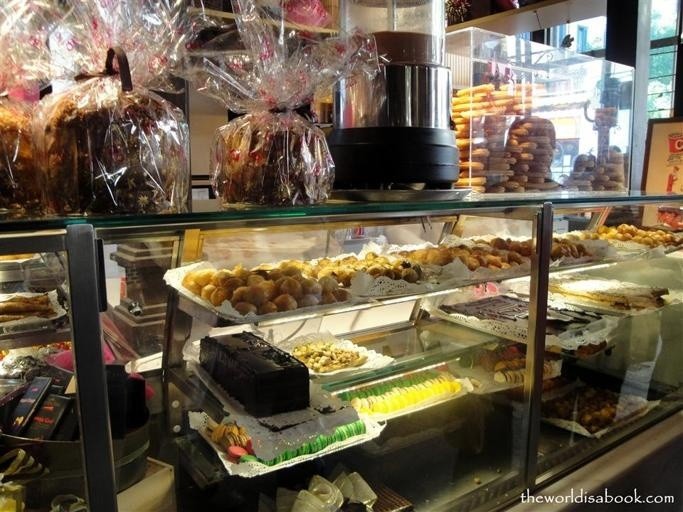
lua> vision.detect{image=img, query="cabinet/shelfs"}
[1,194,682,512]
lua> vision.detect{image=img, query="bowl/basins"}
[0,408,151,508]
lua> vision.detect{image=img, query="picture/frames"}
[636,116,682,233]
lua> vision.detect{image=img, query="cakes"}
[199,274,683,466]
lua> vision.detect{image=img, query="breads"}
[181,222,683,321]
[0,253,55,327]
[43,79,190,214]
[0,103,49,217]
[210,106,334,207]
[447,81,626,194]
